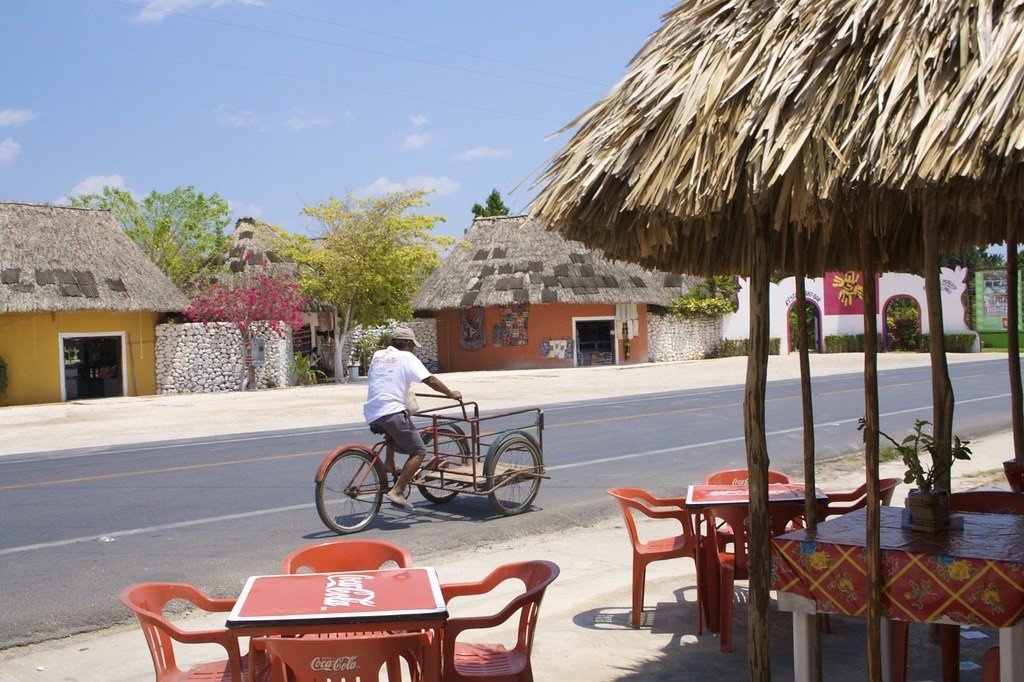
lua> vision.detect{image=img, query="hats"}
[391,326,422,347]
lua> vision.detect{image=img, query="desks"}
[685,483,828,637]
[226,566,448,682]
[771,505,1024,682]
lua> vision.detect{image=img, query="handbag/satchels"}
[408,384,420,416]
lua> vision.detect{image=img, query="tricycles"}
[313,391,552,536]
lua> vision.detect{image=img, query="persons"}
[310,347,321,381]
[364,325,462,511]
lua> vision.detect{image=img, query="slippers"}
[386,496,415,511]
[384,463,400,471]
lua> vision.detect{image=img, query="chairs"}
[703,468,803,552]
[278,538,414,638]
[705,503,829,653]
[951,492,1024,516]
[607,487,708,628]
[440,561,560,682]
[117,582,266,682]
[252,631,430,682]
[824,477,904,517]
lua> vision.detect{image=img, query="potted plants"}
[859,415,971,533]
